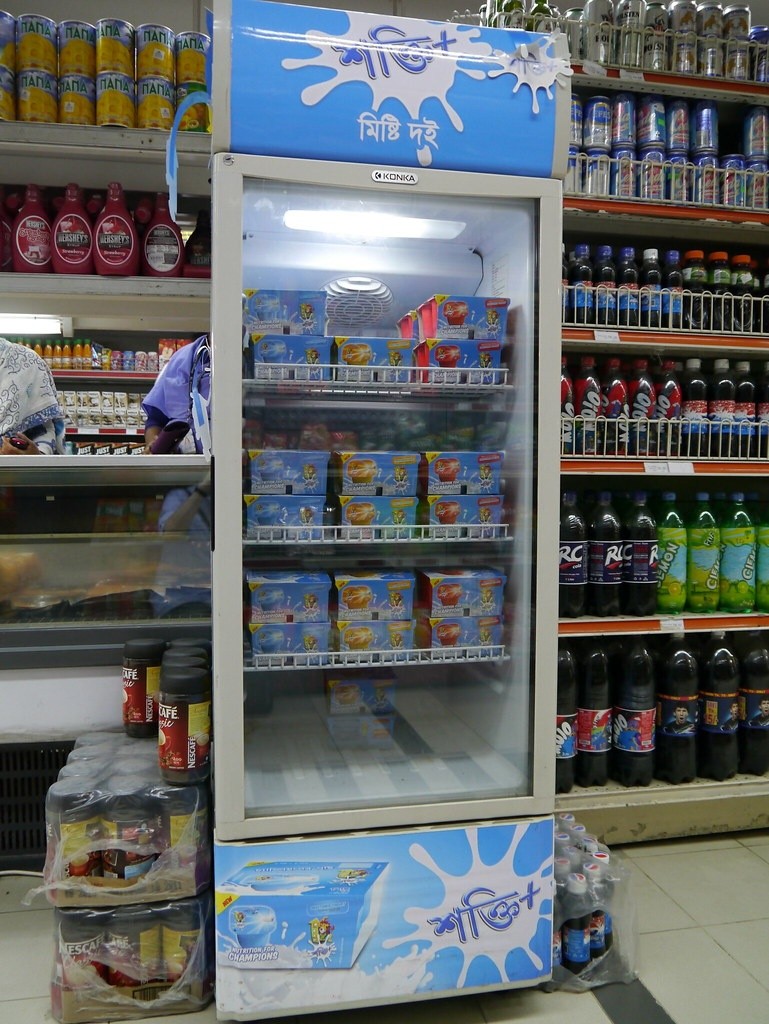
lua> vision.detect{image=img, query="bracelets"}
[195,487,207,498]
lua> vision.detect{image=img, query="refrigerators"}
[208,0,571,1024]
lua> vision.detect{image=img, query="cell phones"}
[9,437,28,449]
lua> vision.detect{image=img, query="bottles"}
[553,489,769,794]
[561,355,769,458]
[41,637,213,989]
[0,181,210,278]
[561,242,769,333]
[539,814,616,994]
[4,337,92,370]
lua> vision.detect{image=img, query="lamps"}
[0,312,74,338]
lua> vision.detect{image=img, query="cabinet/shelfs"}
[0,118,215,488]
[553,60,769,813]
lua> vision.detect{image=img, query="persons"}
[150,470,212,617]
[0,337,66,455]
[142,333,212,454]
